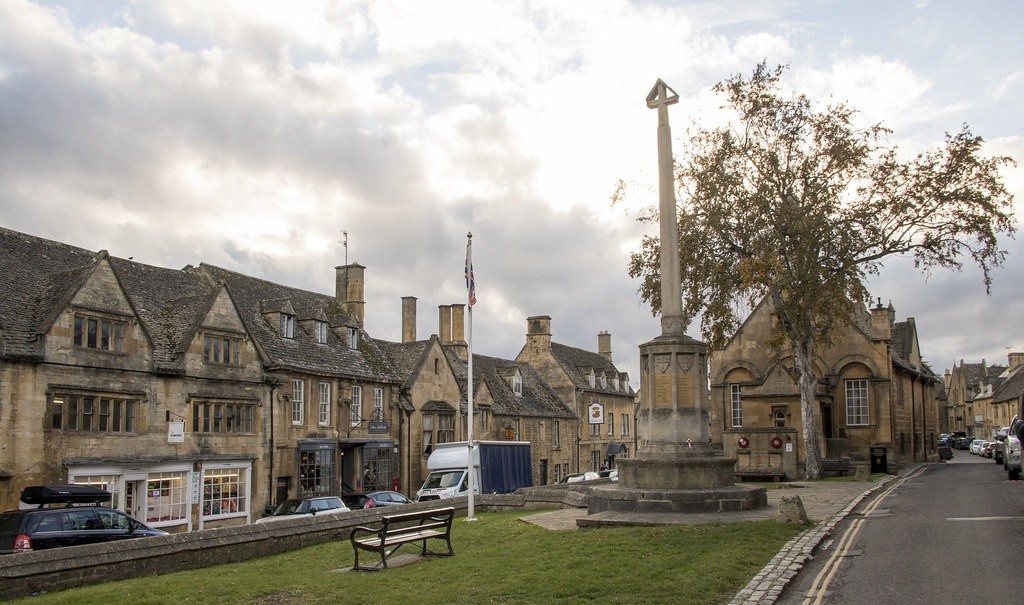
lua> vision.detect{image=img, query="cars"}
[938,430,995,459]
[556,470,614,486]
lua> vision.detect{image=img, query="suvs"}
[341,490,415,513]
[998,414,1023,480]
[0,484,170,554]
[255,496,351,524]
[994,425,1011,464]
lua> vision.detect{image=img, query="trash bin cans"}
[870,446,888,473]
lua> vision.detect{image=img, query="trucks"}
[413,440,536,504]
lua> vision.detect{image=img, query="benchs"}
[349,506,456,570]
[804,461,849,477]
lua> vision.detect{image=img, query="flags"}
[465,244,477,305]
